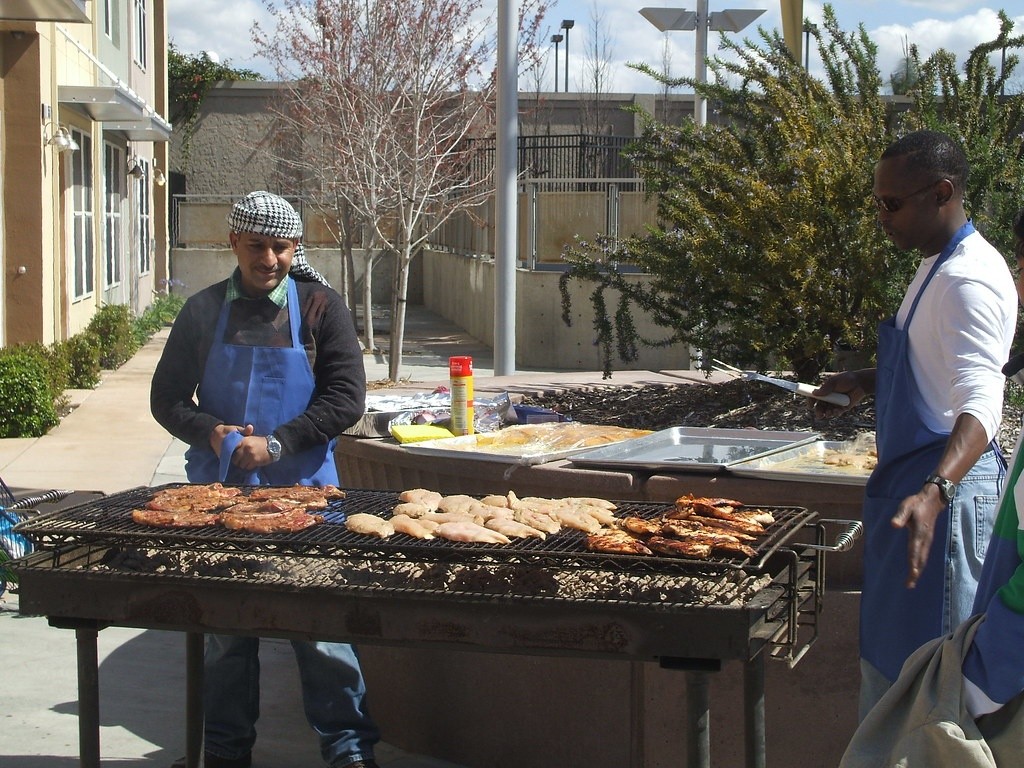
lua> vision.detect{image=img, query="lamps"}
[42,121,70,152]
[126,159,146,180]
[153,168,167,187]
[53,126,80,156]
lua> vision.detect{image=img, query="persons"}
[150,190,379,768]
[962,211,1024,721]
[808,130,1019,725]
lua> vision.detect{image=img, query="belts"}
[981,444,994,454]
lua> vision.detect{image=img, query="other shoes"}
[345,758,380,768]
[171,751,252,768]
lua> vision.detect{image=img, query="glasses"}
[873,176,956,212]
[1011,266,1024,279]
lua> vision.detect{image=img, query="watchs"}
[925,474,957,503]
[266,434,282,463]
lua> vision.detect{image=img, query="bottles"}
[449,356,474,437]
